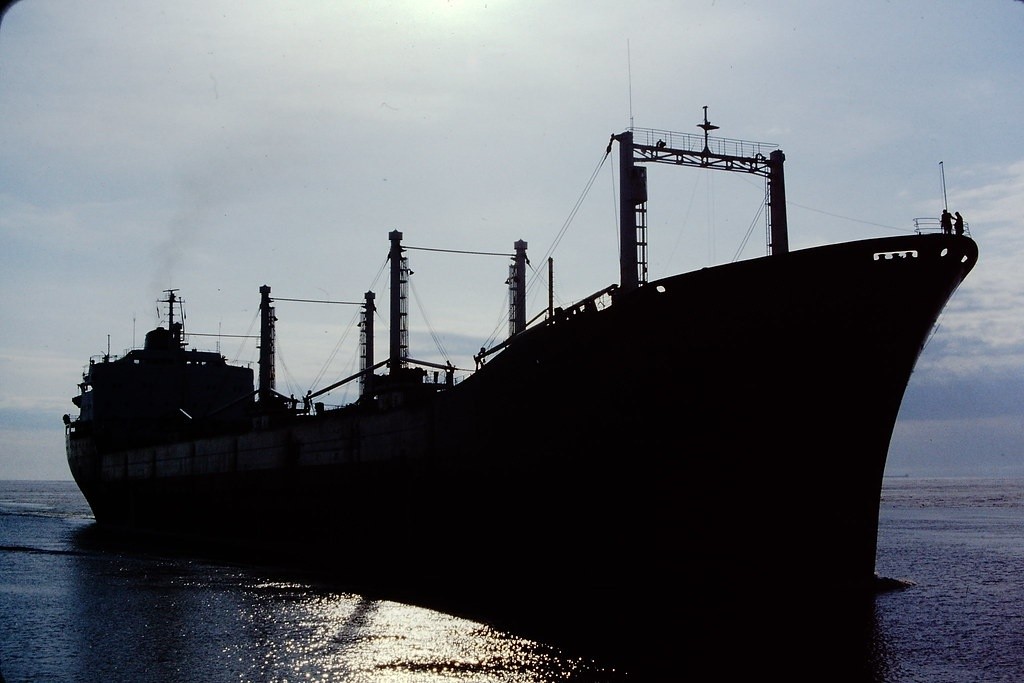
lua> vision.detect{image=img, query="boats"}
[63,43,980,642]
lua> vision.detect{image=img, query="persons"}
[940,210,954,234]
[954,212,963,235]
